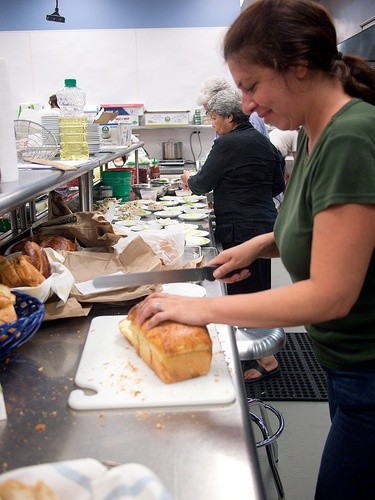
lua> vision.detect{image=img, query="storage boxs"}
[93,103,190,152]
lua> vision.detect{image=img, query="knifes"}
[92,265,243,289]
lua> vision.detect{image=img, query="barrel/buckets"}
[163,138,183,159]
[101,167,135,197]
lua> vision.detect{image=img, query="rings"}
[157,302,161,309]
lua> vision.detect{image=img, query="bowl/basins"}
[10,272,56,303]
[166,183,178,195]
[132,184,168,201]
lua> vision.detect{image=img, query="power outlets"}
[192,127,202,134]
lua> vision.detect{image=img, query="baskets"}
[0,291,45,357]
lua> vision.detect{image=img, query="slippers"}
[244,361,282,384]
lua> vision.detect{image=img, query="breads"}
[118,302,212,384]
[9,234,77,251]
[0,240,51,287]
[0,283,21,341]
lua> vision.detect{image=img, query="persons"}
[196,75,282,205]
[181,88,286,383]
[49,94,59,109]
[270,126,300,157]
[132,0,375,500]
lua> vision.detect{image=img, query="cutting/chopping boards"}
[68,315,237,411]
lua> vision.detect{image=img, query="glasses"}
[205,111,210,116]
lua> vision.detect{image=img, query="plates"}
[101,146,128,153]
[178,214,208,221]
[159,196,207,210]
[113,210,152,221]
[143,206,163,211]
[153,211,183,218]
[184,209,213,214]
[41,115,102,155]
[112,219,211,247]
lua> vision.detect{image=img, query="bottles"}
[150,157,161,179]
[56,78,89,161]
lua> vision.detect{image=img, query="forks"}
[175,189,193,196]
